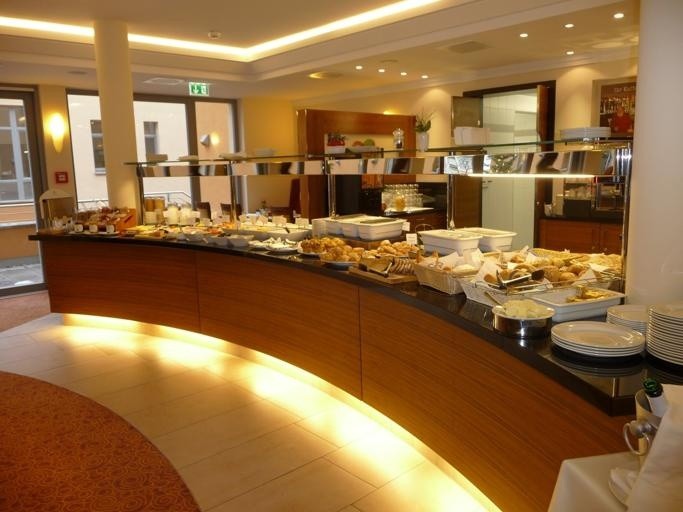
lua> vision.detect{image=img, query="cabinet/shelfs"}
[534,178,625,255]
[298,108,415,223]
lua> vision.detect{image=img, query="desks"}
[367,207,445,234]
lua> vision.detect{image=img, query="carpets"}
[0,371,201,512]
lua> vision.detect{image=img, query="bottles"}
[640,377,671,419]
[392,127,403,150]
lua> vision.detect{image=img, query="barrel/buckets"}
[621,389,662,469]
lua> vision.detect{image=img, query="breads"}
[301,235,618,319]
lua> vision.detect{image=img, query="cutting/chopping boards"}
[348,256,417,285]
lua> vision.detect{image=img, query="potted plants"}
[415,110,436,152]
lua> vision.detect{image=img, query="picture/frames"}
[589,75,637,138]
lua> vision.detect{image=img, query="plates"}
[548,300,683,368]
[559,126,614,140]
[68,231,120,236]
[346,145,380,153]
[247,241,358,268]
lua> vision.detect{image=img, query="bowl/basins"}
[490,305,558,339]
[253,149,274,157]
[143,210,313,249]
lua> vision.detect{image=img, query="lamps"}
[48,111,66,154]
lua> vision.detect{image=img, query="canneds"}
[74,220,84,233]
[106,223,115,233]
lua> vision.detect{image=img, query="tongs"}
[357,257,396,277]
[493,267,544,290]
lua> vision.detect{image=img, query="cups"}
[105,223,115,233]
[73,222,84,233]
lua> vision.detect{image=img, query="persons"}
[608,106,632,134]
